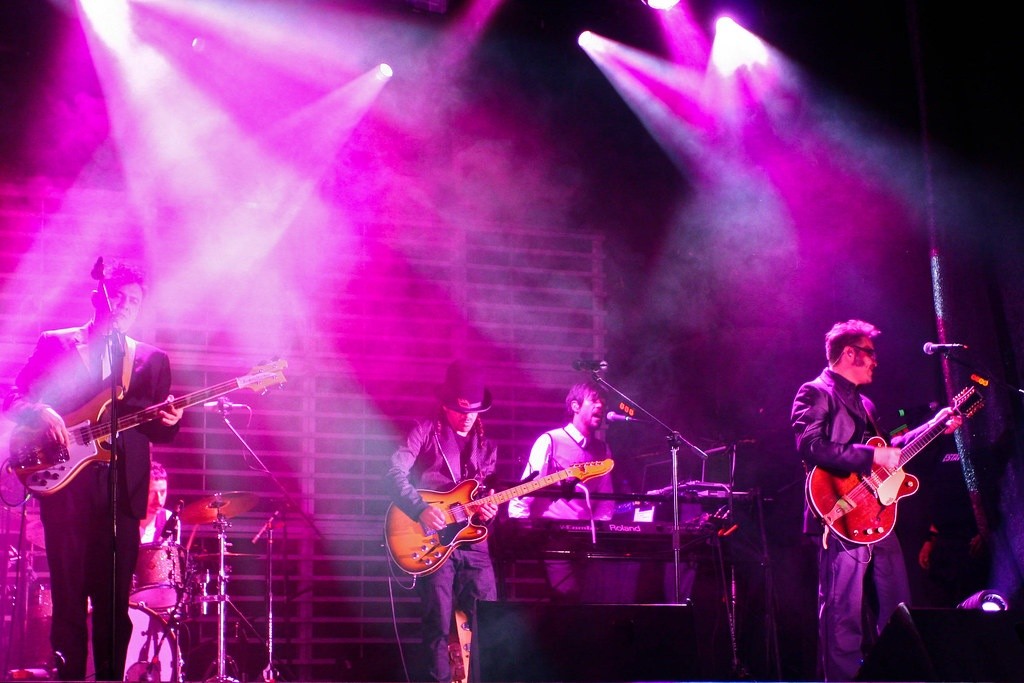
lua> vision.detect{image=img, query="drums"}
[86,602,187,683]
[129,544,188,612]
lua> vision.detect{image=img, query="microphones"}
[572,359,608,371]
[922,342,970,354]
[91,256,103,280]
[607,411,650,423]
[203,401,247,412]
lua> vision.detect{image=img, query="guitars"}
[8,359,289,497]
[805,386,986,545]
[383,459,615,573]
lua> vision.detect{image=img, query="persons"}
[791,319,963,683]
[123,459,185,683]
[507,380,632,604]
[13,255,190,683]
[384,359,498,683]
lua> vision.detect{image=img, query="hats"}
[434,361,493,414]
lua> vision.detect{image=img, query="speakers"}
[852,602,1024,683]
[467,597,701,683]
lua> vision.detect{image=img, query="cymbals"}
[193,552,262,562]
[178,493,262,525]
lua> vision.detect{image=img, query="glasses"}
[853,345,879,358]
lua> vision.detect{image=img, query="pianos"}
[492,515,742,564]
